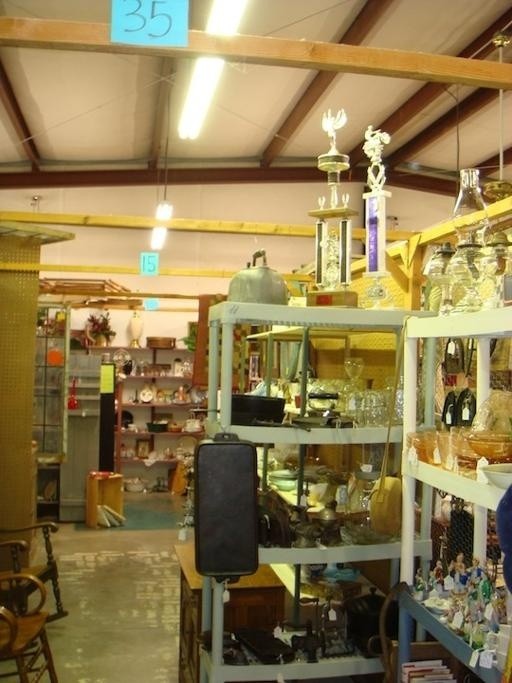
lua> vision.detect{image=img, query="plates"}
[140,389,153,402]
[268,468,297,491]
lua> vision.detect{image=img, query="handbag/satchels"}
[369,476,401,535]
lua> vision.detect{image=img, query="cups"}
[298,356,402,426]
[484,621,509,670]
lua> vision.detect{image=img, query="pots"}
[344,587,402,655]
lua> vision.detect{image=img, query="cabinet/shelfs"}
[115,347,208,500]
[33,303,102,522]
[200,301,433,683]
[398,308,512,683]
[174,544,286,682]
[192,295,269,392]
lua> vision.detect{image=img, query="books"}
[402,660,457,683]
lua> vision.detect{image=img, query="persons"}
[433,552,506,652]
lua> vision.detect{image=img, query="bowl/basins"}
[146,423,167,433]
[464,432,512,465]
[479,463,512,490]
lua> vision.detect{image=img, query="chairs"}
[0,574,58,683]
[0,522,68,622]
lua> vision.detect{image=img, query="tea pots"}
[228,248,290,306]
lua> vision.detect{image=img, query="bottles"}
[463,591,504,660]
[419,233,512,318]
[454,167,486,216]
